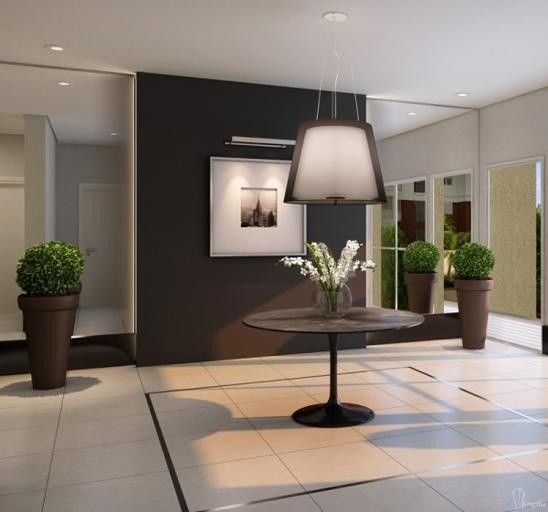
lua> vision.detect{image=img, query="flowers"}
[280,239,375,283]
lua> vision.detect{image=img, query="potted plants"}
[401,240,440,313]
[14,240,84,392]
[449,242,495,350]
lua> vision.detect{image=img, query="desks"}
[241,305,426,428]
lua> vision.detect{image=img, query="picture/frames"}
[208,156,308,257]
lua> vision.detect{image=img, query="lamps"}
[285,9,389,205]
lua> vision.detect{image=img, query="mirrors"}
[365,96,480,319]
[485,154,545,324]
[0,61,139,343]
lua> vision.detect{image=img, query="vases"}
[309,283,352,317]
